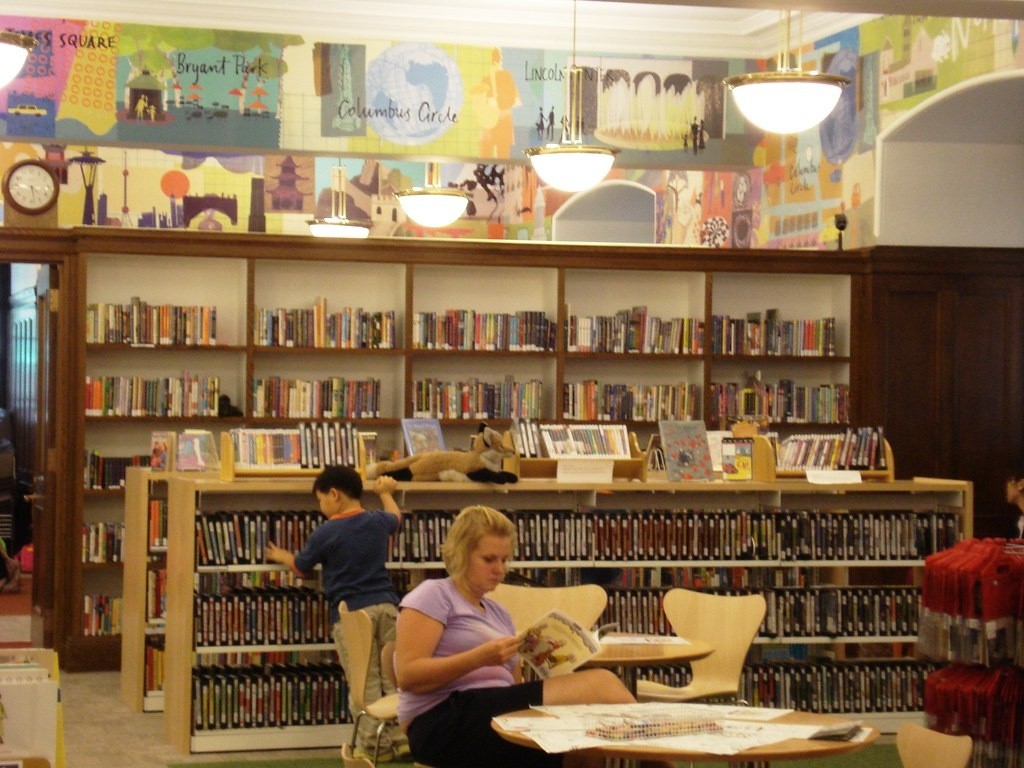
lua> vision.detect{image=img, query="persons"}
[263,463,411,763]
[393,506,675,768]
[1005,475,1024,540]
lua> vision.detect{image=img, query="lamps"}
[305,164,375,237]
[720,8,850,135]
[521,1,616,192]
[393,160,469,227]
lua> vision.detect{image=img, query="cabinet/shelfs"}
[121,432,898,714]
[162,475,973,757]
[59,224,867,668]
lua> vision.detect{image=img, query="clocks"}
[1,158,60,216]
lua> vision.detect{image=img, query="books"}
[1,296,965,768]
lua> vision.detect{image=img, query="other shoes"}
[393,740,411,757]
[353,742,395,762]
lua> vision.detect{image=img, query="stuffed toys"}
[366,428,519,484]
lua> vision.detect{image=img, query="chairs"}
[483,582,608,632]
[895,723,973,768]
[635,588,768,703]
[333,601,406,768]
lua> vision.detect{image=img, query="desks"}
[488,707,880,768]
[583,631,714,698]
